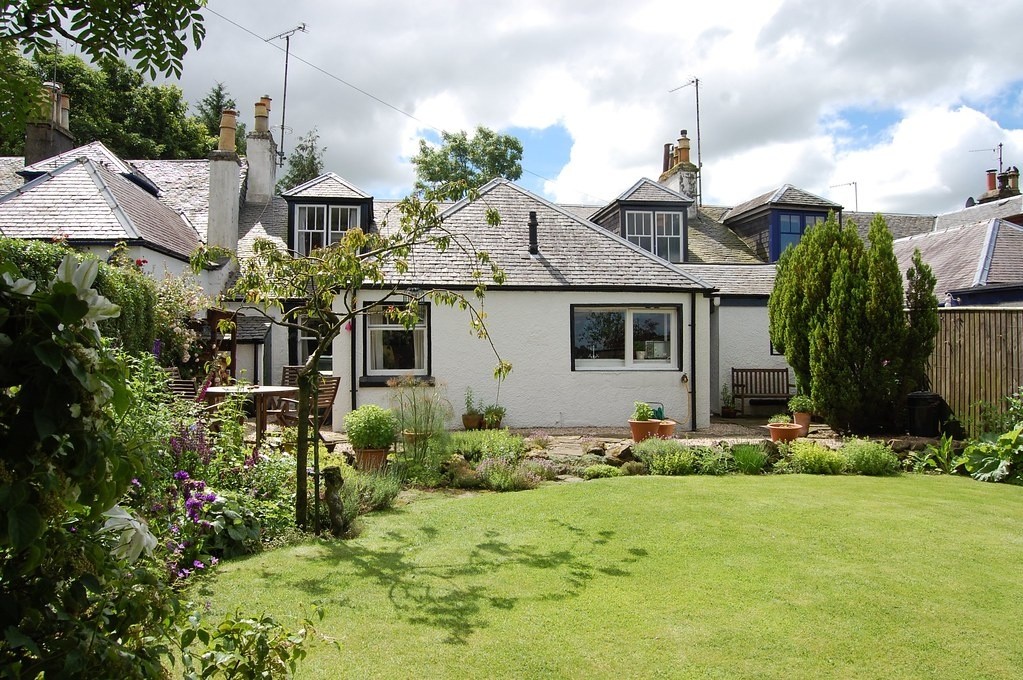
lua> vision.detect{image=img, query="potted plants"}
[482,404,507,430]
[628,401,661,444]
[787,394,814,437]
[462,385,482,430]
[344,404,399,473]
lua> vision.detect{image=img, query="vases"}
[657,420,676,439]
[767,422,803,446]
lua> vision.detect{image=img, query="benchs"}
[731,366,797,418]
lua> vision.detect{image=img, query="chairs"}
[282,374,341,432]
[265,365,307,416]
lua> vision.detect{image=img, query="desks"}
[203,384,300,450]
